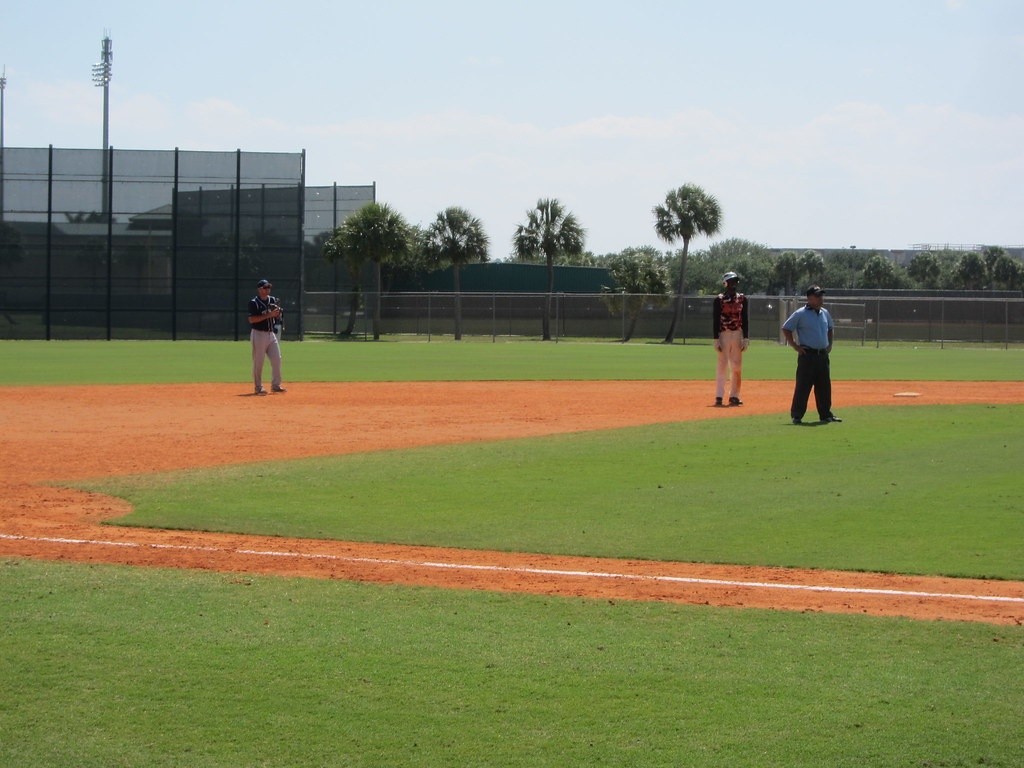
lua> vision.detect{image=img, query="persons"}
[249,280,285,395]
[713,272,750,406]
[782,285,843,424]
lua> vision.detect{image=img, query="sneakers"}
[255,386,268,396]
[821,416,842,422]
[715,397,723,405]
[271,385,287,392]
[729,397,743,404]
[793,418,801,424]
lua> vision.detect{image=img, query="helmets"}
[722,272,739,287]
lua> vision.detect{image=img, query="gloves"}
[741,338,750,352]
[713,340,723,352]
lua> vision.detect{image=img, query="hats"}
[807,284,824,296]
[257,279,272,288]
